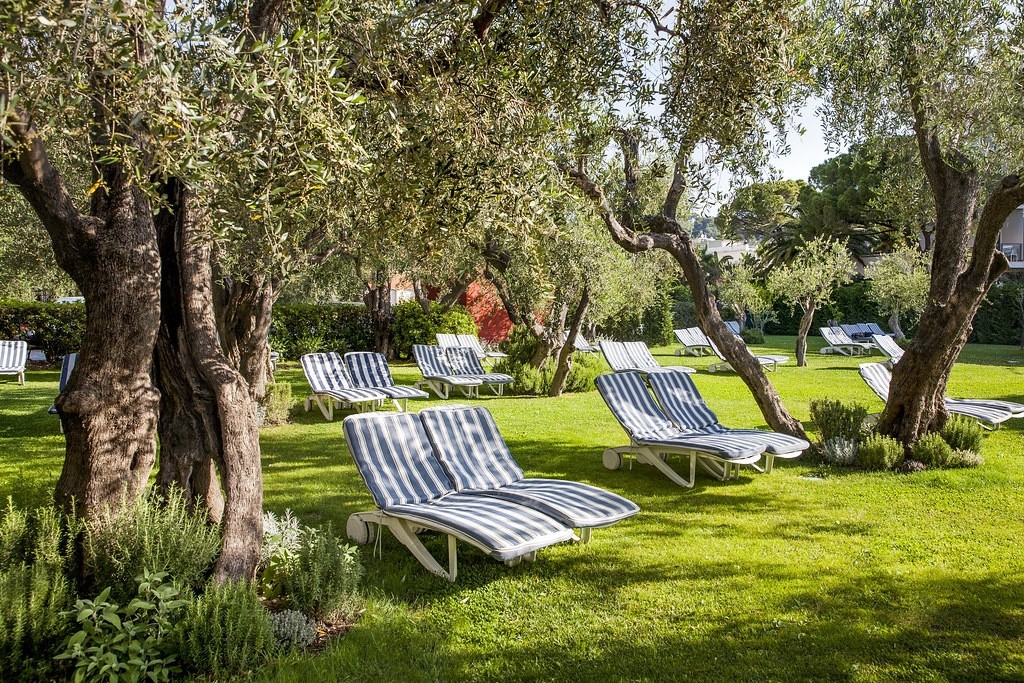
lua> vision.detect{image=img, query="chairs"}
[818,322,905,363]
[890,357,1024,419]
[0,339,28,387]
[436,333,487,362]
[686,326,716,356]
[46,352,80,434]
[859,362,1012,433]
[593,371,767,492]
[672,328,715,357]
[412,344,483,401]
[564,329,602,358]
[343,351,430,413]
[623,341,697,375]
[648,371,813,480]
[419,406,641,544]
[300,352,388,421]
[706,321,792,373]
[341,413,584,583]
[457,334,510,366]
[446,347,515,396]
[598,341,674,388]
